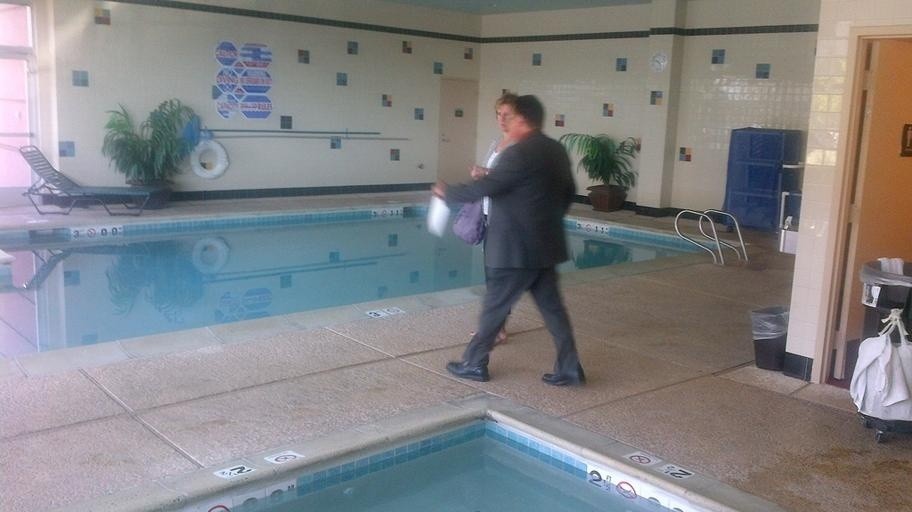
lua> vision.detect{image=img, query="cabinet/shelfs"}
[724,126,805,237]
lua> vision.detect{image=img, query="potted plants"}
[558,131,637,213]
[102,97,198,210]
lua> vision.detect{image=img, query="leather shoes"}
[542,372,585,387]
[445,361,490,382]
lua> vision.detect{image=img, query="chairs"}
[0,243,158,294]
[18,144,153,216]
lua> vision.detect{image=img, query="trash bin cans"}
[849,257,912,444]
[751,305,790,370]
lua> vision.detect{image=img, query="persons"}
[431,93,590,387]
[470,92,520,346]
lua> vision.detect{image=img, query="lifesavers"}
[191,237,230,276]
[189,139,228,179]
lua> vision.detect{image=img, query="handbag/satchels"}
[452,199,486,247]
